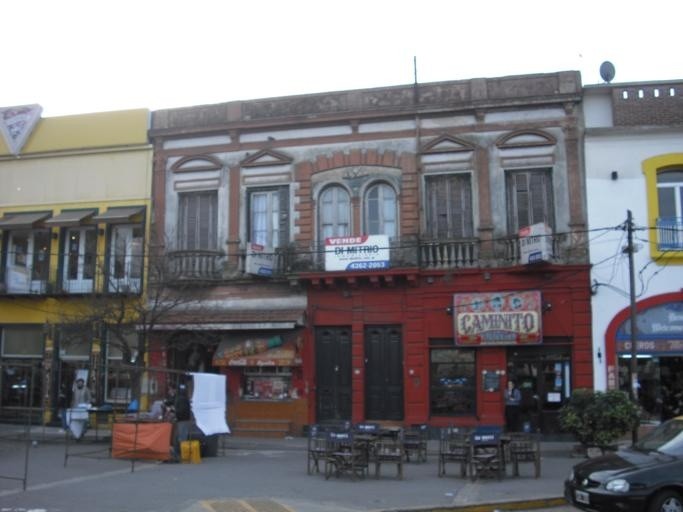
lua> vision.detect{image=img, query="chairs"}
[307,421,541,483]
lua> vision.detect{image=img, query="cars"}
[561,414,683,512]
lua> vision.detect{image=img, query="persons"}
[503,380,522,432]
[163,384,190,422]
[72,378,91,406]
